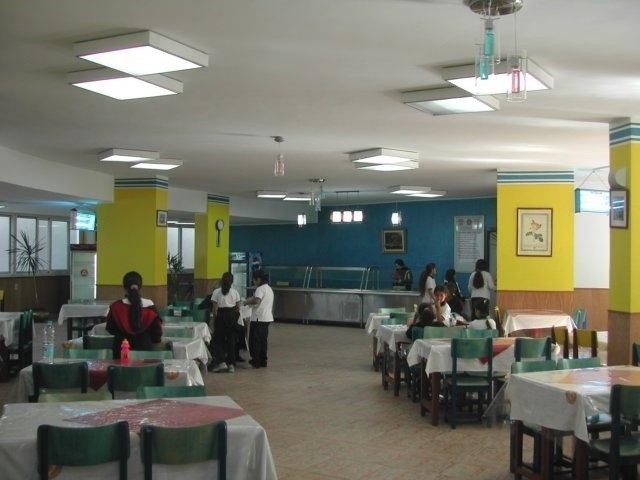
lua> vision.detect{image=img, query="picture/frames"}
[516,206,553,257]
[380,228,407,254]
[609,188,629,229]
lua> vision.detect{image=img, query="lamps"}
[441,58,553,96]
[75,31,209,77]
[478,6,500,64]
[401,87,500,115]
[474,43,494,89]
[357,161,419,172]
[274,143,285,176]
[282,193,318,201]
[67,68,184,102]
[332,193,342,222]
[129,160,183,171]
[406,190,445,198]
[342,192,352,222]
[505,8,528,102]
[99,149,161,162]
[390,186,431,194]
[352,148,419,165]
[257,192,286,198]
[353,192,362,222]
[391,196,401,225]
[297,203,306,224]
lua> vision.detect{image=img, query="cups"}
[407,318,414,327]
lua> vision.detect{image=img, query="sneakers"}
[212,361,235,373]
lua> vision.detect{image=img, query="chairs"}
[35,420,131,480]
[376,306,607,403]
[495,337,551,425]
[573,385,639,479]
[1,299,250,400]
[510,360,591,478]
[558,357,631,478]
[444,338,492,429]
[140,419,228,480]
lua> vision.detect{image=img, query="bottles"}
[120,339,130,364]
[169,305,173,315]
[40,320,56,364]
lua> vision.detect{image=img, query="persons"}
[243,270,275,369]
[210,272,240,373]
[105,271,163,360]
[391,258,413,291]
[406,258,497,402]
[197,281,246,362]
